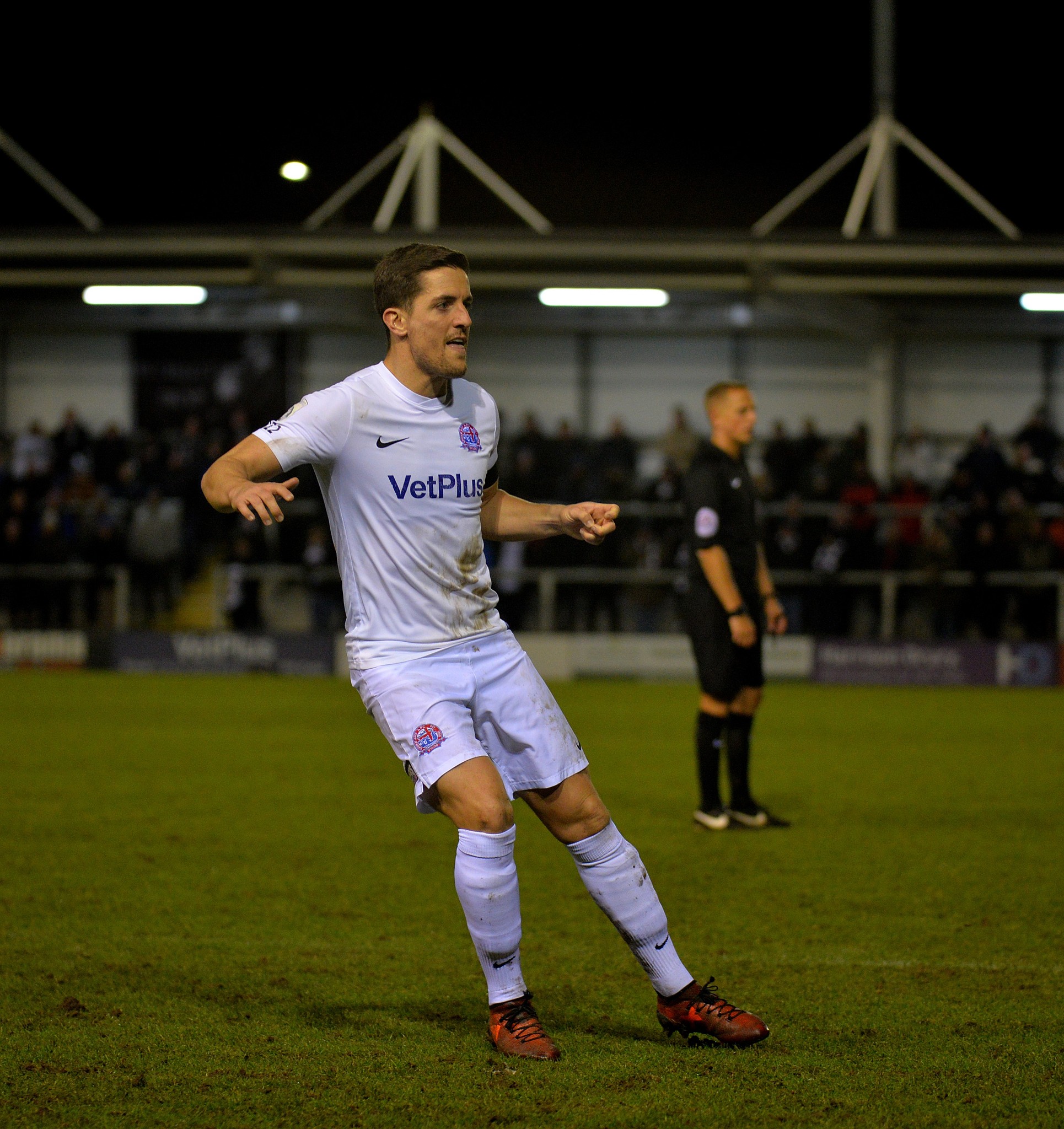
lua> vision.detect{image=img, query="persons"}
[199,242,775,1058]
[2,380,1062,830]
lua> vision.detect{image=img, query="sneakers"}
[487,990,562,1061]
[657,975,769,1046]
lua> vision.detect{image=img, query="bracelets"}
[762,589,778,599]
[724,602,748,618]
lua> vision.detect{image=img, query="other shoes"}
[692,809,740,830]
[728,804,791,828]
